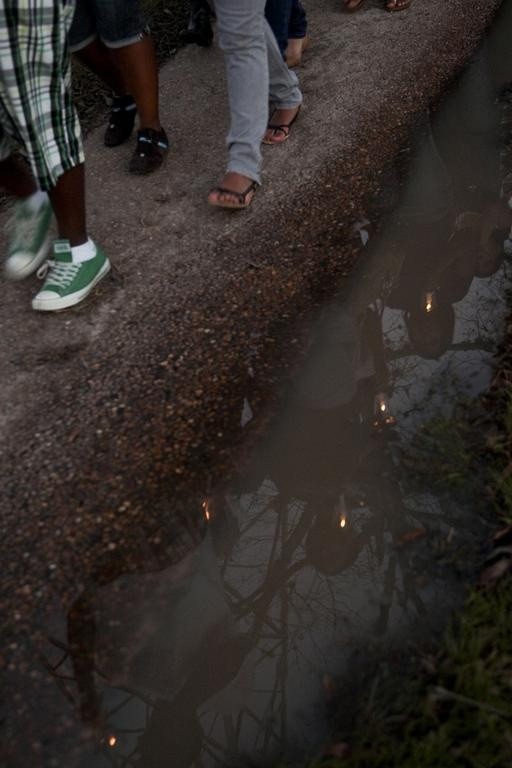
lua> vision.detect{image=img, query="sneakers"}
[2,191,53,282]
[30,238,113,311]
[104,96,135,146]
[130,124,167,173]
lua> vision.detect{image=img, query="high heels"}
[284,35,315,66]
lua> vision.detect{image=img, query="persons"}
[66,0,172,176]
[203,0,303,210]
[336,1,416,11]
[266,0,313,69]
[0,0,111,313]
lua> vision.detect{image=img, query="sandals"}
[207,173,252,213]
[264,102,302,146]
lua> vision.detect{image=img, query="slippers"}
[339,1,411,12]
